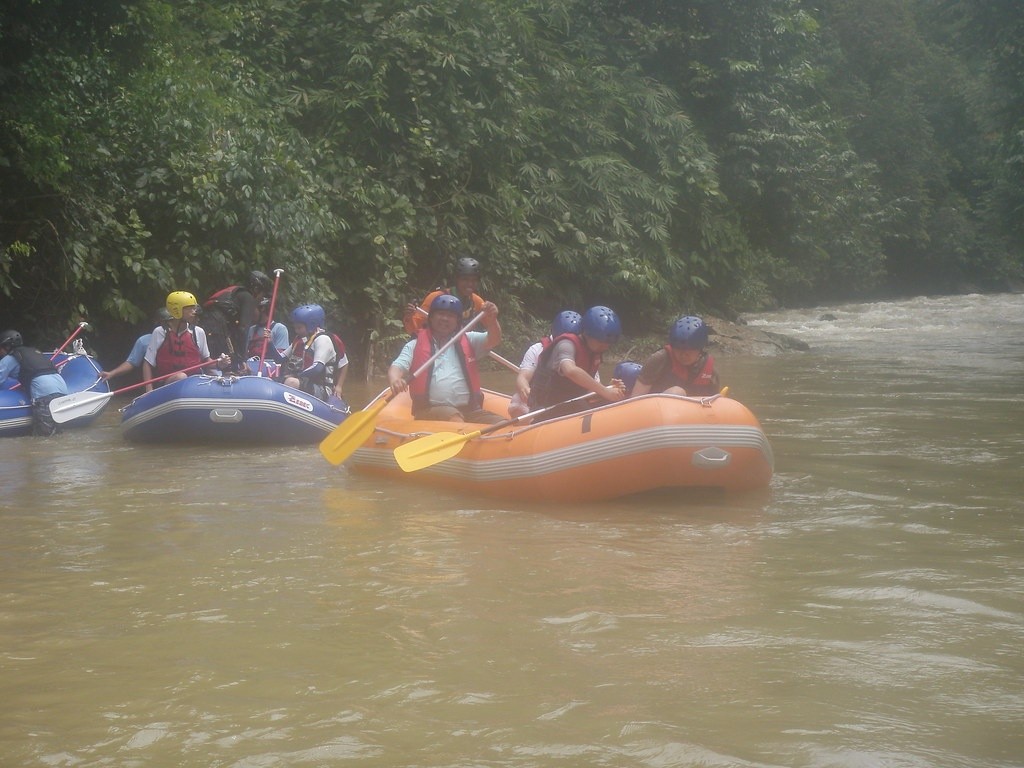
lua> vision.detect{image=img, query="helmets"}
[551,310,584,338]
[430,294,463,316]
[167,291,198,319]
[256,296,270,314]
[0,329,22,350]
[154,307,173,326]
[613,363,642,396]
[250,270,274,298]
[292,305,325,334]
[670,316,706,352]
[454,257,482,282]
[584,307,619,344]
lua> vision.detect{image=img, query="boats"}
[0,352,112,435]
[117,374,352,443]
[345,386,772,510]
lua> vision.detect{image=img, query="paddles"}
[392,383,618,474]
[10,356,76,390]
[48,354,228,424]
[317,306,487,466]
[256,268,285,378]
[407,296,522,375]
[49,321,89,362]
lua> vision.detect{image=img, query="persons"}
[402,258,482,339]
[508,306,643,424]
[631,315,721,398]
[388,295,508,425]
[98,270,348,410]
[0,330,67,436]
